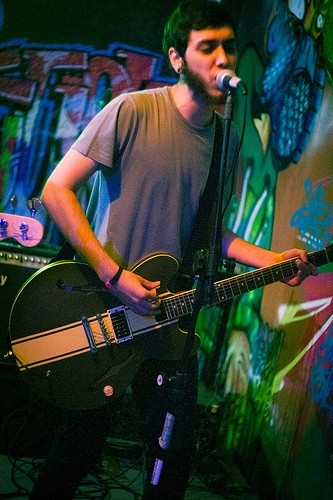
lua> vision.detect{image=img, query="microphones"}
[216,70,248,95]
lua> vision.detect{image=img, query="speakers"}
[0,241,60,382]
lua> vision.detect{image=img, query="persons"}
[27,0,317,500]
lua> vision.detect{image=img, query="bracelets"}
[102,266,122,289]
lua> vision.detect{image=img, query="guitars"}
[9,243,333,412]
